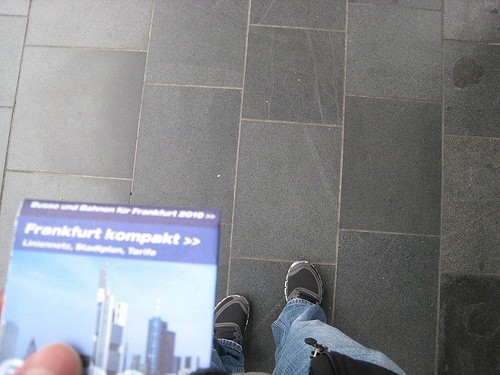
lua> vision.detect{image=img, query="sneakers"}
[213,294,251,348]
[284,260,324,307]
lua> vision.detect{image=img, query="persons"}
[6,261,408,375]
